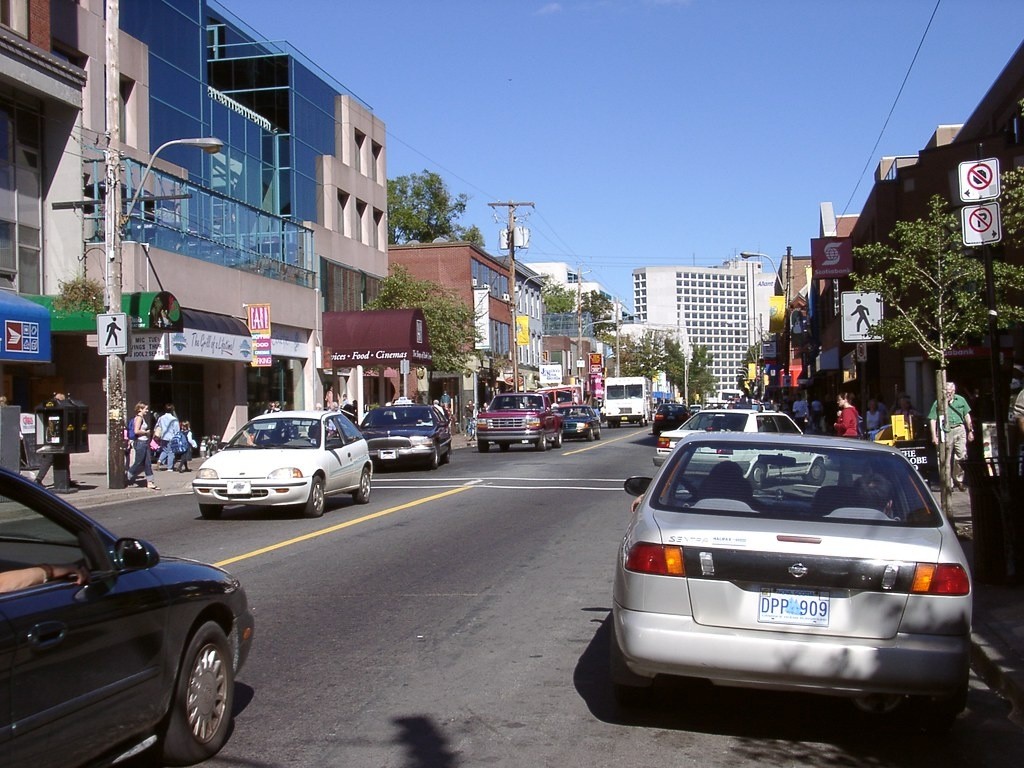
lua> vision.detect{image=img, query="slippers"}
[151,486,160,490]
[127,481,139,487]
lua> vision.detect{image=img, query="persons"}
[793,393,809,434]
[867,393,918,432]
[440,390,451,405]
[264,401,281,413]
[834,392,859,437]
[927,382,974,492]
[154,402,197,473]
[812,396,823,428]
[581,408,585,413]
[35,394,73,487]
[342,393,349,408]
[313,402,341,436]
[434,399,449,421]
[464,399,474,433]
[306,424,320,440]
[126,401,161,490]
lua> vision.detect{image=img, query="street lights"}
[105,137,224,489]
[740,252,793,412]
[577,319,615,382]
[511,274,548,394]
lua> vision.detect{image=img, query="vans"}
[605,376,651,428]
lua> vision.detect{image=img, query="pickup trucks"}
[473,392,564,452]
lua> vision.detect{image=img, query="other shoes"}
[177,466,183,473]
[955,480,966,492]
[168,469,173,471]
[157,460,162,471]
[34,478,44,488]
[185,468,192,472]
[950,486,953,492]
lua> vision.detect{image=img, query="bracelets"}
[970,429,973,432]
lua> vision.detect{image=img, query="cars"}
[0,464,254,768]
[556,405,601,442]
[358,397,453,471]
[652,404,726,434]
[192,411,374,520]
[653,409,828,490]
[613,431,973,711]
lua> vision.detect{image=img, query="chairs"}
[682,498,757,512]
[826,507,899,521]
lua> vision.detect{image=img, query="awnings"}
[0,288,51,363]
[322,308,433,427]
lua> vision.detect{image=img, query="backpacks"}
[170,430,190,454]
[127,414,143,440]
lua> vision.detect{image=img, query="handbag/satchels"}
[857,414,863,437]
